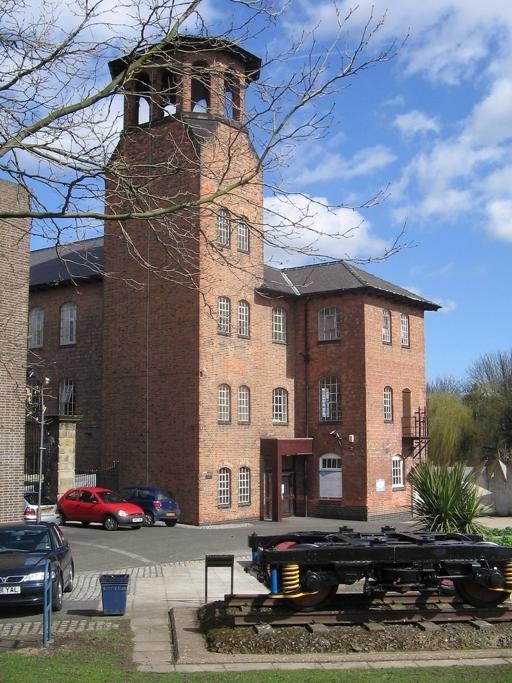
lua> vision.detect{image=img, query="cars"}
[57,487,147,530]
[25,490,61,526]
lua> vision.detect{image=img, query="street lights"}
[36,376,52,524]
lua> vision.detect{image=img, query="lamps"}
[330,430,343,450]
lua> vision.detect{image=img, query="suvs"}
[0,521,75,613]
[114,486,182,526]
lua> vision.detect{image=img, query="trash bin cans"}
[100,574,129,616]
